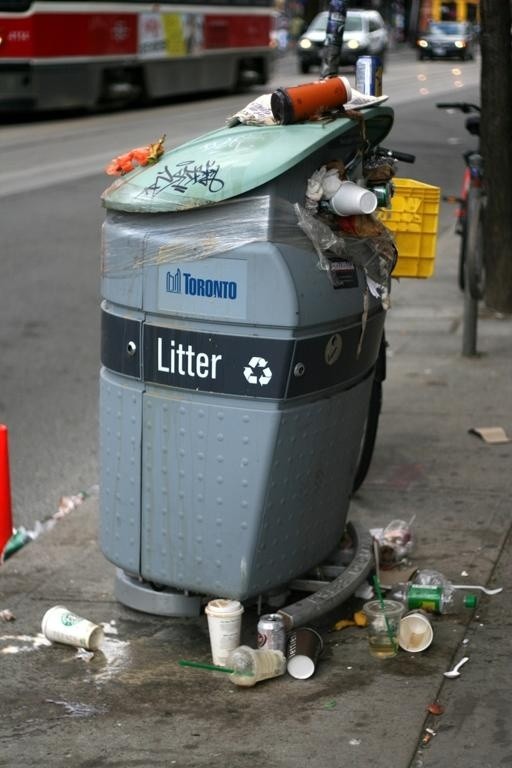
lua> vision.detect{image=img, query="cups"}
[40,606,105,655]
[271,75,352,128]
[201,598,245,667]
[398,606,436,653]
[224,644,287,687]
[363,601,405,659]
[289,627,326,679]
[328,177,380,219]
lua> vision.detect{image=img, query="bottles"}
[380,519,413,564]
[388,583,479,619]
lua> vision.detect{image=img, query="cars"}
[416,21,478,62]
[297,8,389,76]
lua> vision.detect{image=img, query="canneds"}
[257,614,286,658]
[355,55,382,98]
[368,183,392,206]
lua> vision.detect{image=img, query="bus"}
[0,0,278,127]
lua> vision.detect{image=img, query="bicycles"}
[437,101,487,357]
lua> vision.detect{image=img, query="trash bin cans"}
[98,104,389,628]
